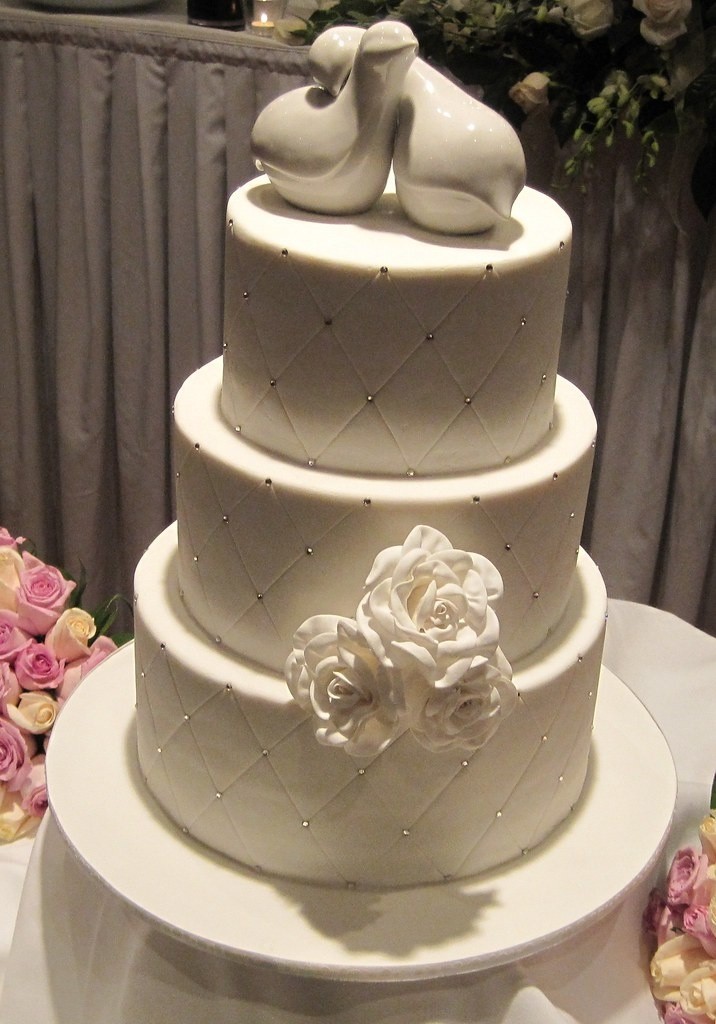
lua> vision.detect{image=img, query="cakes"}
[130,22,607,891]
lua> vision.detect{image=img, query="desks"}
[0,2,715,652]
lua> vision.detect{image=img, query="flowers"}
[0,524,132,846]
[283,521,516,762]
[640,807,715,1023]
